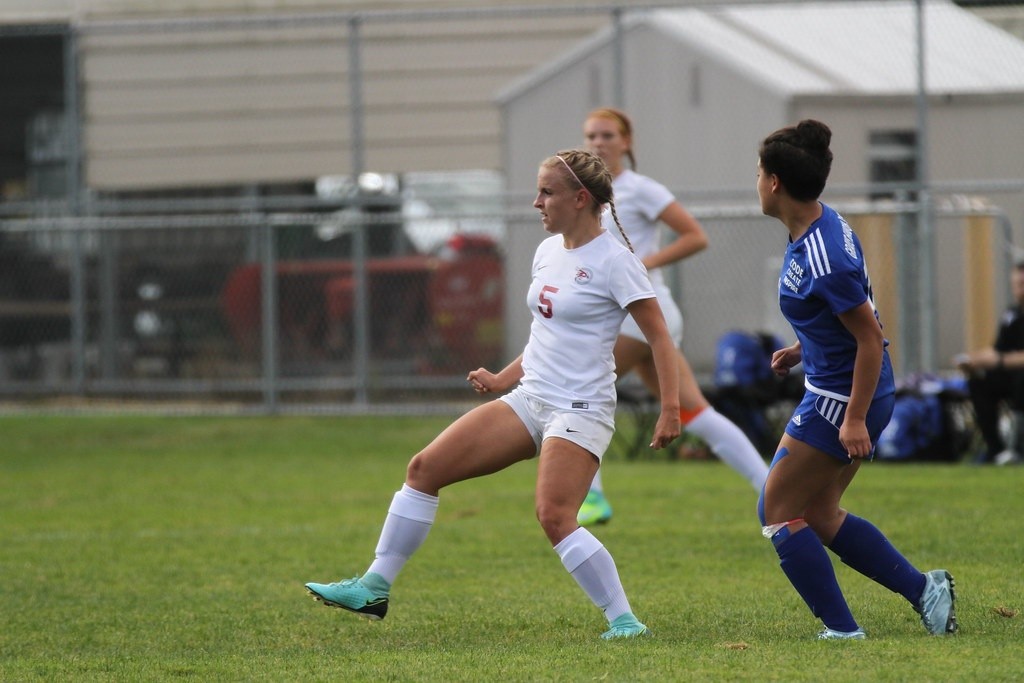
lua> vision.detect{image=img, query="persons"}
[756,121,961,646]
[296,104,778,644]
[959,260,1024,467]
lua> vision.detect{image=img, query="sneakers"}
[817,624,866,642]
[576,490,612,526]
[597,624,650,640]
[304,574,389,621]
[911,570,958,638]
